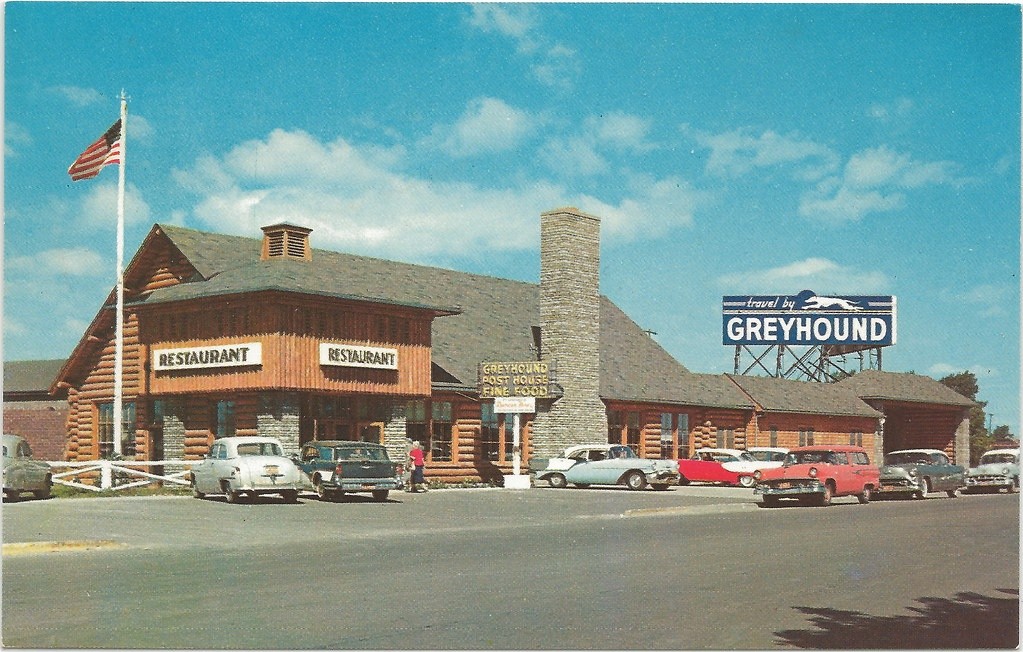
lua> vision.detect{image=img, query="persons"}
[410,441,429,493]
[706,453,713,461]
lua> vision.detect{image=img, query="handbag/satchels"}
[407,459,416,470]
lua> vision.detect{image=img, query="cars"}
[751,444,880,507]
[676,447,798,489]
[867,448,966,498]
[293,440,405,503]
[191,436,313,499]
[962,447,1022,495]
[0,434,54,501]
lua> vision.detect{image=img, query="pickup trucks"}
[535,443,682,492]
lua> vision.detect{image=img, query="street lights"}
[988,413,994,436]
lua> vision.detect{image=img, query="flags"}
[68,119,122,181]
